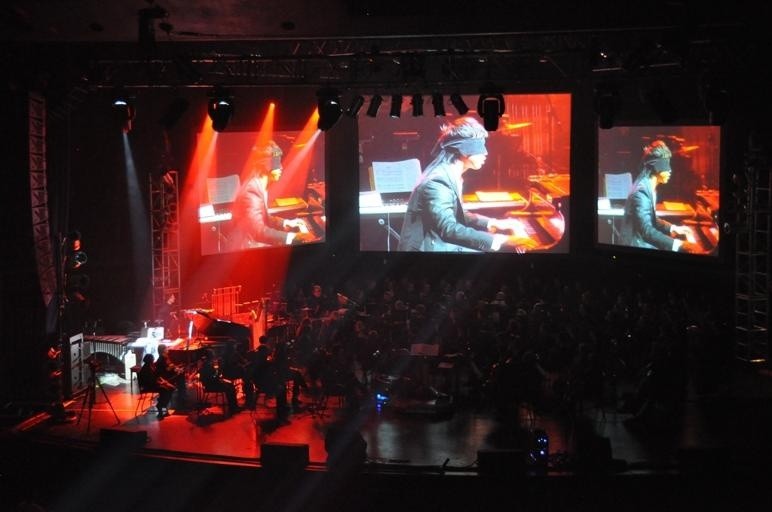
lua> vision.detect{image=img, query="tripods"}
[77,367,120,433]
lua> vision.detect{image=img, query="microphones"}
[337,293,348,300]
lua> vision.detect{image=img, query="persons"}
[225,138,320,249]
[139,261,728,439]
[615,138,704,255]
[390,115,538,255]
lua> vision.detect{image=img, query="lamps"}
[91,50,729,131]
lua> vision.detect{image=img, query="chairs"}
[129,323,620,427]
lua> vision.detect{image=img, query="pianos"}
[285,182,325,245]
[166,310,252,372]
[505,173,570,254]
[672,190,720,254]
